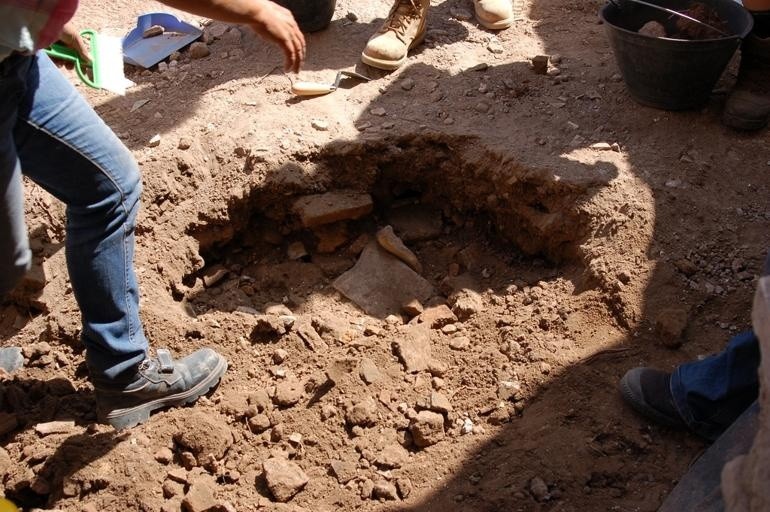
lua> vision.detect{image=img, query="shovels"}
[291,71,371,96]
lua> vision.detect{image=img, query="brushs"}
[43,30,125,96]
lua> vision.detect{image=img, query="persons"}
[720,0,770,130]
[619,331,761,430]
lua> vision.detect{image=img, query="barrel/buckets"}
[598,0,754,112]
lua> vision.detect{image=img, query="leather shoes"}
[94,348,228,431]
[473,0,514,30]
[360,0,430,71]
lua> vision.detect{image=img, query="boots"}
[724,34,769,130]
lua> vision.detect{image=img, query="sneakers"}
[619,367,680,427]
